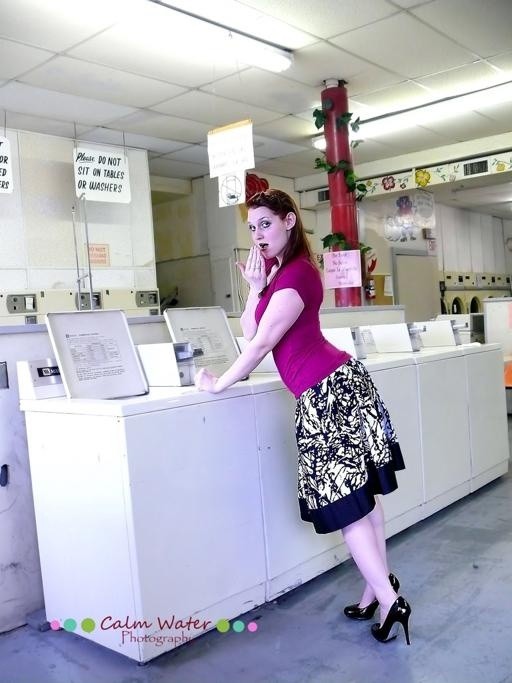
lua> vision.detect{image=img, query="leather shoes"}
[343,573,400,621]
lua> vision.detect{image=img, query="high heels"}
[370,595,413,647]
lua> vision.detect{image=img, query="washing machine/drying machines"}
[15,270,512,663]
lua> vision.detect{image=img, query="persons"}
[190,186,411,645]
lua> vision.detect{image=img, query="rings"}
[255,265,260,269]
